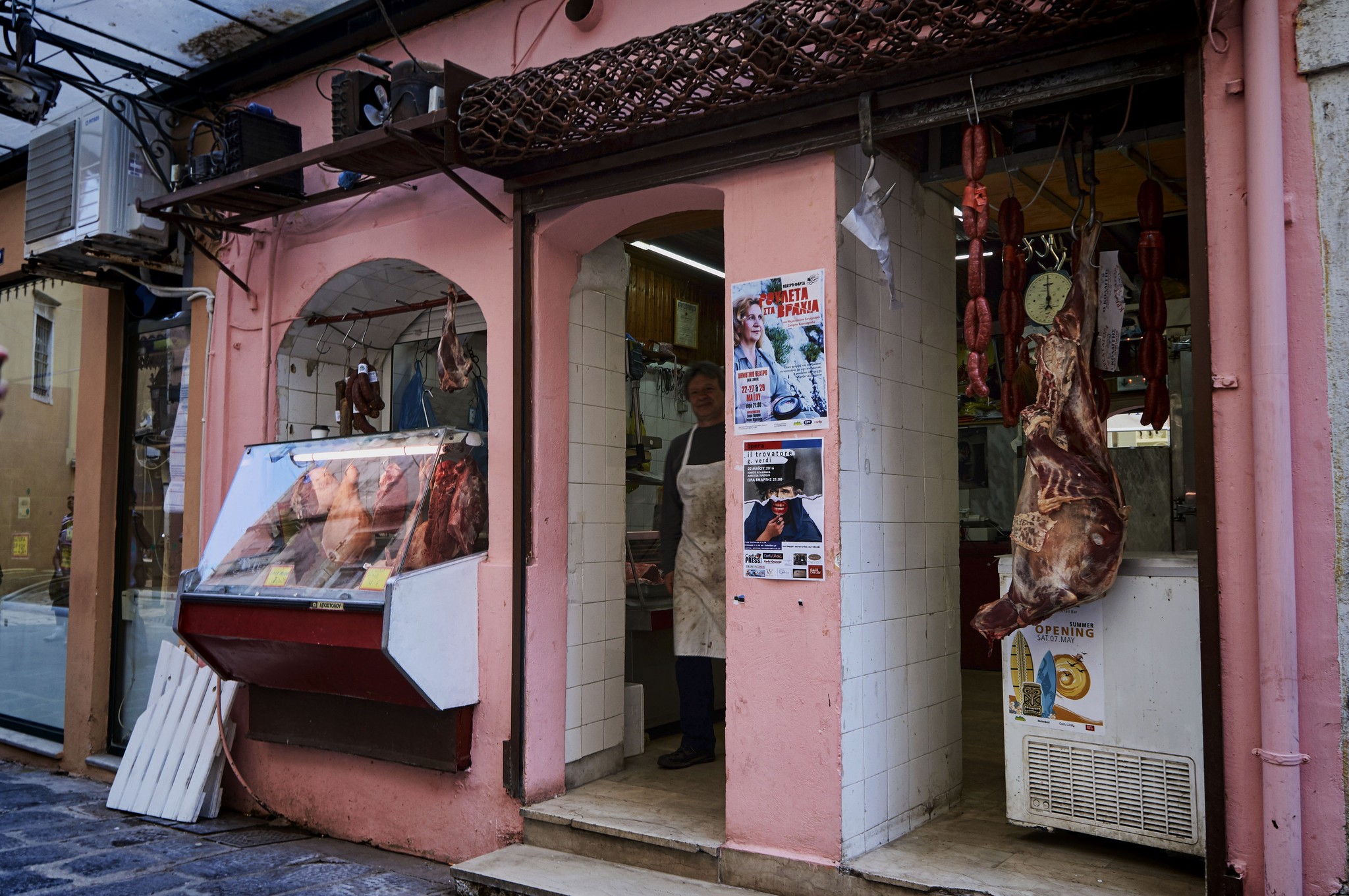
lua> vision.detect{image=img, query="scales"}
[1025,261,1075,330]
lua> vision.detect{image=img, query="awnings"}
[0,1,503,191]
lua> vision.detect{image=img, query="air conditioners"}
[23,90,174,259]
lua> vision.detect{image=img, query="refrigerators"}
[0,568,178,746]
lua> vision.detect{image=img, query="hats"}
[755,456,803,494]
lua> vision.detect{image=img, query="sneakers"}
[42,628,65,641]
[657,741,715,769]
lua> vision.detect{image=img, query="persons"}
[745,456,822,542]
[732,295,821,425]
[656,360,726,769]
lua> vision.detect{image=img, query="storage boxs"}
[181,423,490,774]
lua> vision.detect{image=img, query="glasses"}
[67,496,75,500]
[129,501,137,506]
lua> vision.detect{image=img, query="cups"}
[310,425,330,439]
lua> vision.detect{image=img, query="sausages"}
[1139,178,1169,431]
[998,195,1027,428]
[335,357,385,436]
[961,125,990,397]
